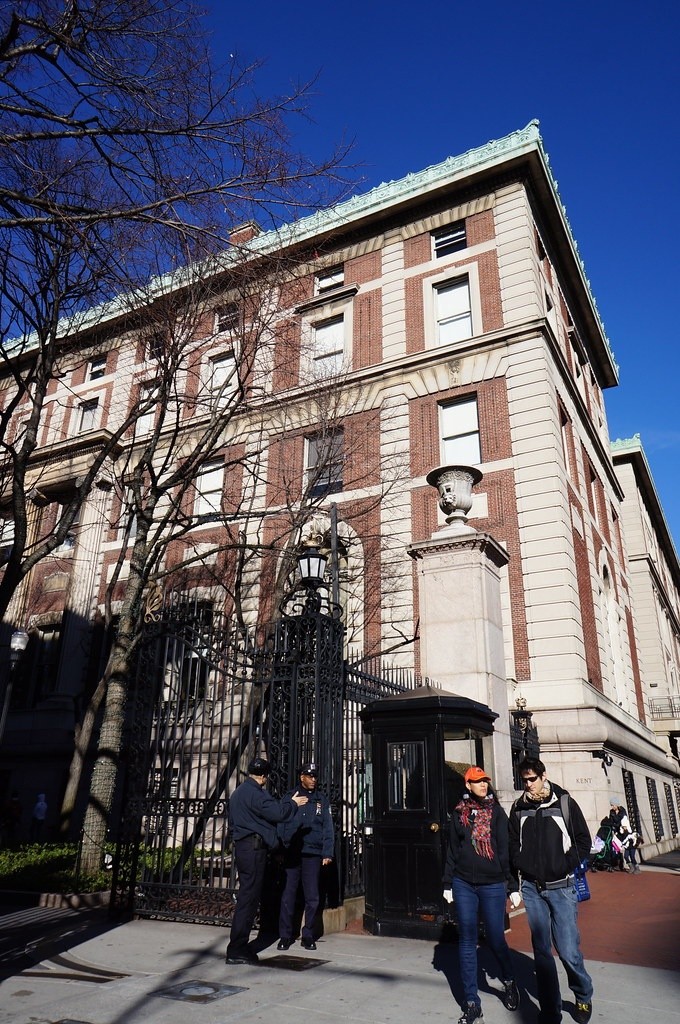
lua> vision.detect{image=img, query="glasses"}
[302,773,319,780]
[522,775,539,783]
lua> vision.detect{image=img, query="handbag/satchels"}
[611,834,622,851]
[573,858,591,902]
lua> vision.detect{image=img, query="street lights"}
[0,627,29,744]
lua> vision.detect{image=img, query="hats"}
[610,798,619,805]
[620,816,633,834]
[300,763,318,775]
[464,766,491,782]
[248,758,272,776]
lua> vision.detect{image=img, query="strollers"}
[588,826,626,873]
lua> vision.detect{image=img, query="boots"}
[634,864,640,874]
[627,862,635,874]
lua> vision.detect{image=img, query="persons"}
[0,790,23,840]
[589,797,644,874]
[443,767,521,1024]
[28,793,48,840]
[226,757,308,965]
[508,757,594,1024]
[277,764,334,950]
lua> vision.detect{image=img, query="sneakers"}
[226,951,258,964]
[539,1012,563,1024]
[576,999,592,1024]
[257,934,278,943]
[458,1001,483,1024]
[504,976,521,1011]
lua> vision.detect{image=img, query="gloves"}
[443,889,454,904]
[509,892,522,907]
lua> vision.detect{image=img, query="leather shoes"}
[301,936,316,950]
[277,936,295,949]
